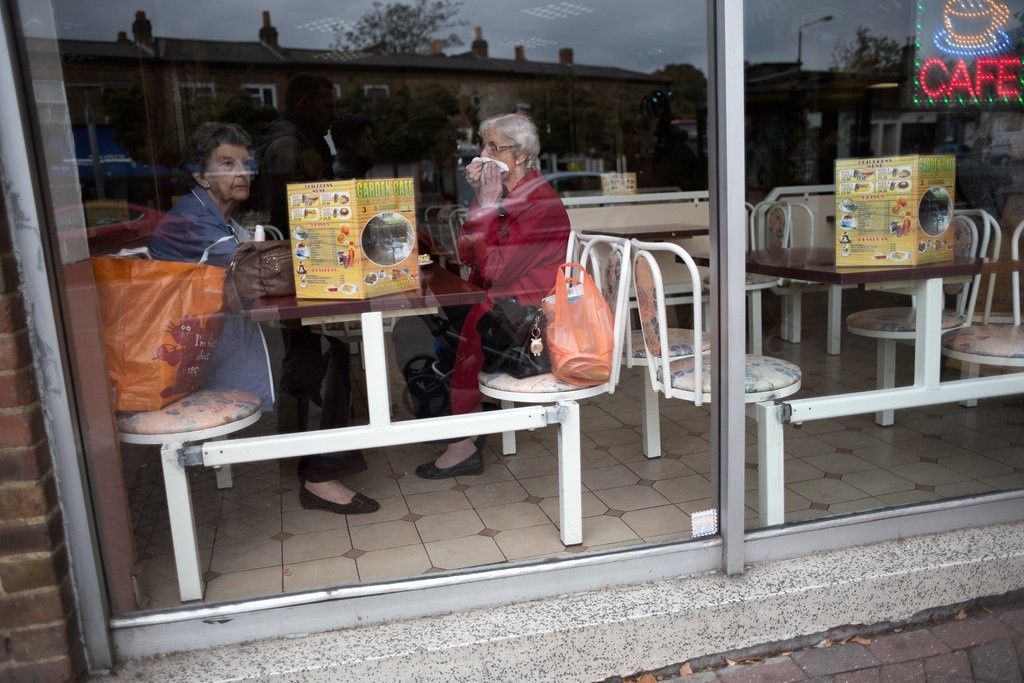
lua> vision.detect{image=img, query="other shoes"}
[299,482,379,514]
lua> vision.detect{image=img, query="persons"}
[410,112,573,483]
[261,70,358,482]
[142,120,384,516]
[326,111,378,180]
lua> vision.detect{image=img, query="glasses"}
[474,142,519,155]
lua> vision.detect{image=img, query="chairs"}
[476,231,631,545]
[92,245,265,604]
[587,234,715,460]
[749,200,841,362]
[627,238,802,528]
[841,207,1023,428]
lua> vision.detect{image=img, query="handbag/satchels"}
[540,262,613,389]
[236,239,294,300]
[476,295,550,379]
[91,233,242,415]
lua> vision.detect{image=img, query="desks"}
[159,257,586,603]
[678,235,1023,529]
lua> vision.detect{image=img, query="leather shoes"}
[414,449,484,480]
